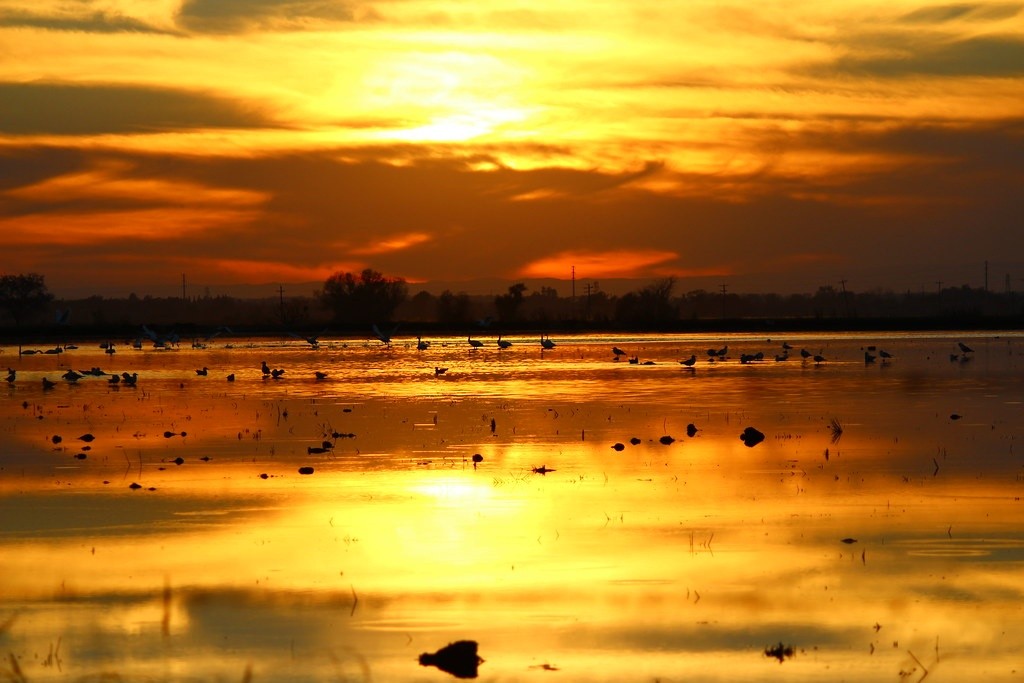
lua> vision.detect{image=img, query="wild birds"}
[20,337,207,355]
[435,366,448,376]
[372,328,559,353]
[195,361,286,383]
[302,334,328,380]
[6,368,17,382]
[42,368,138,389]
[308,441,335,454]
[611,332,978,367]
